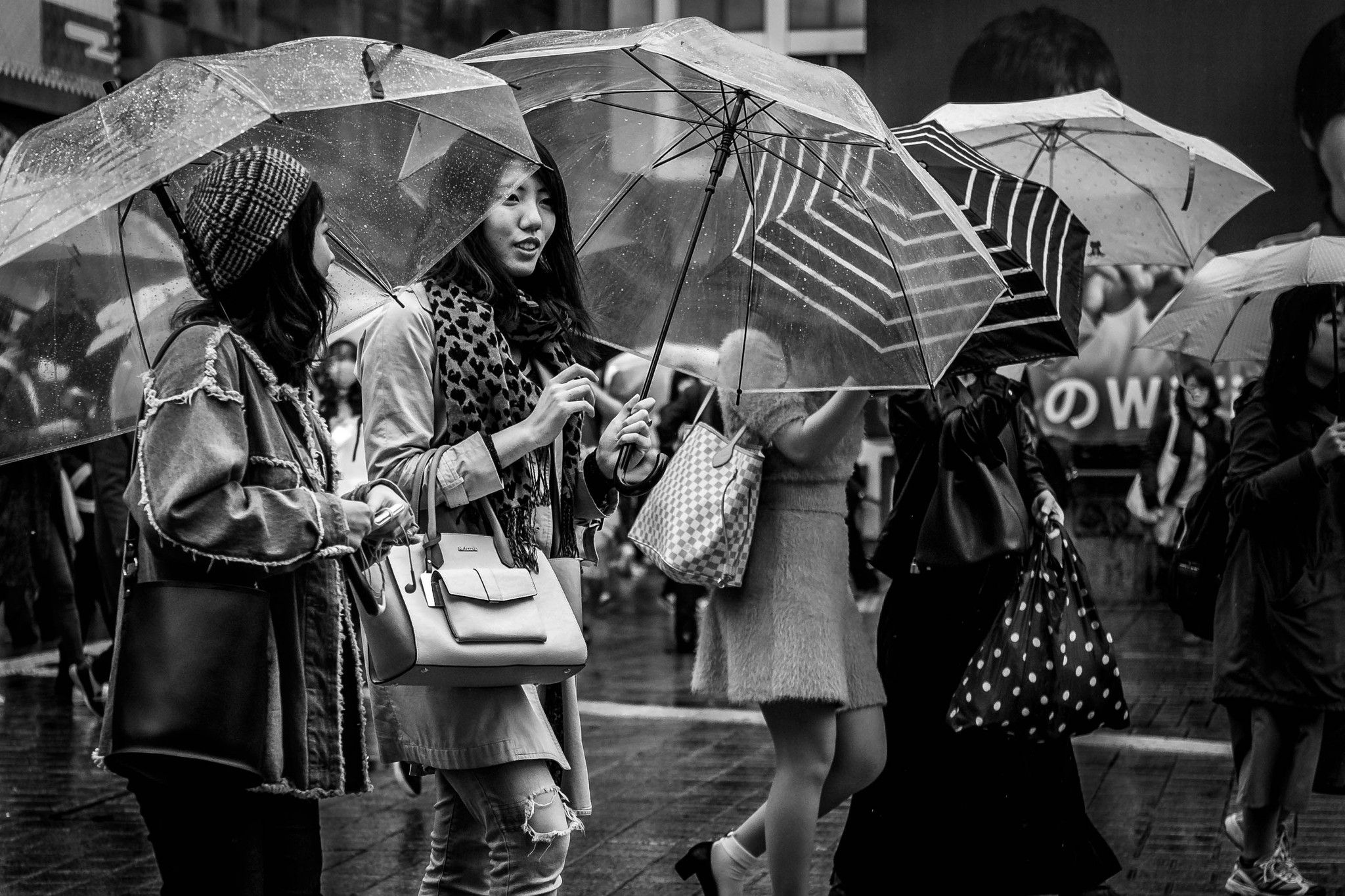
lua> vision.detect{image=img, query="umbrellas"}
[396,18,1006,487]
[85,300,231,419]
[601,347,719,409]
[1128,236,1345,421]
[914,88,1275,273]
[701,119,1092,381]
[0,36,537,616]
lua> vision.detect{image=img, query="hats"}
[180,144,313,298]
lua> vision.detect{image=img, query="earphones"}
[405,570,416,594]
[372,591,386,617]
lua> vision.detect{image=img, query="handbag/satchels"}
[627,379,765,589]
[108,322,269,783]
[349,444,587,686]
[1126,412,1181,524]
[944,512,1130,739]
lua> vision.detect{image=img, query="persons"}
[0,290,200,722]
[364,124,661,896]
[1200,283,1345,896]
[1142,358,1227,591]
[676,220,1118,896]
[98,145,410,896]
[570,366,884,649]
[318,337,368,496]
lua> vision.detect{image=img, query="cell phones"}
[373,503,403,522]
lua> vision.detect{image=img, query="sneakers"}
[1224,811,1325,896]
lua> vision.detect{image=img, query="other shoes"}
[393,757,424,797]
[69,663,104,721]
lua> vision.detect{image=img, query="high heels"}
[676,842,719,896]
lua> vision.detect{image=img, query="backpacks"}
[1171,454,1231,641]
